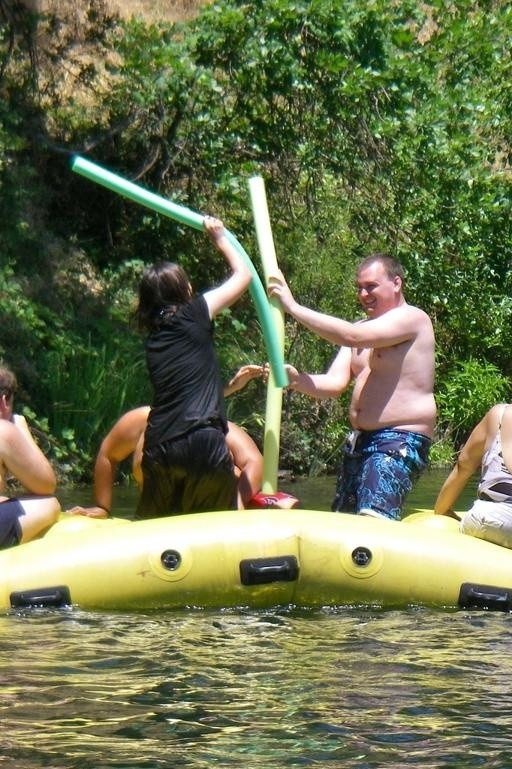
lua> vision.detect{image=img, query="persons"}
[265,252,438,521]
[136,215,264,521]
[433,403,512,550]
[65,404,265,520]
[0,366,62,553]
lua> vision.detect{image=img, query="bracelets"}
[96,505,111,516]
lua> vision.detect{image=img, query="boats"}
[0,502,512,616]
